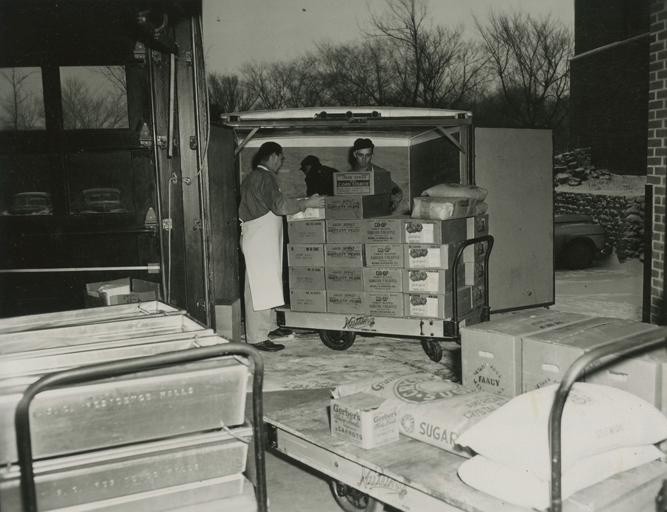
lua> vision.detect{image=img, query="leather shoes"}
[250,340,285,353]
[267,328,293,338]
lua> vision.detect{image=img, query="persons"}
[298,152,342,195]
[346,137,409,217]
[237,140,327,352]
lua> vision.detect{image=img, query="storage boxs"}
[284,172,666,448]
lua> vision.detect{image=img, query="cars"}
[555,214,604,270]
[2,188,128,215]
